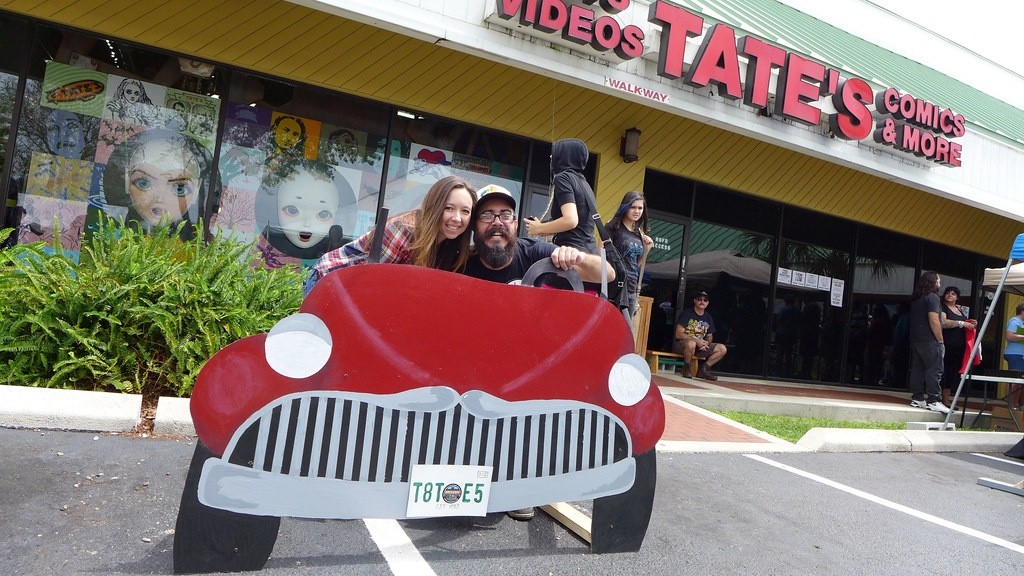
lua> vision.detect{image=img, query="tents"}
[938,233,1024,431]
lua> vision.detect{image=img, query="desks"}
[960,374,1024,432]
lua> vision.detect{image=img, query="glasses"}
[696,297,708,302]
[476,212,516,224]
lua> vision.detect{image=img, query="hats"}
[694,291,708,297]
[476,184,516,211]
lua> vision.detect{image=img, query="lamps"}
[620,126,641,163]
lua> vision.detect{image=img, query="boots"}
[683,363,692,378]
[700,363,717,380]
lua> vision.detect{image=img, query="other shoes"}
[943,402,959,410]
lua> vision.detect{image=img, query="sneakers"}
[926,402,954,414]
[910,400,926,408]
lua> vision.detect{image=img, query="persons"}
[306,175,477,296]
[598,191,653,333]
[906,271,954,414]
[436,184,615,288]
[674,290,727,380]
[523,138,601,298]
[939,286,978,410]
[1004,303,1024,411]
[773,291,910,387]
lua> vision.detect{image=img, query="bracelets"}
[959,321,964,328]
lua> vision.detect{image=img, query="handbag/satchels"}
[604,241,626,300]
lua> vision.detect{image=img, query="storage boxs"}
[990,407,1024,432]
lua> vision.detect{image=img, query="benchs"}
[647,350,706,377]
